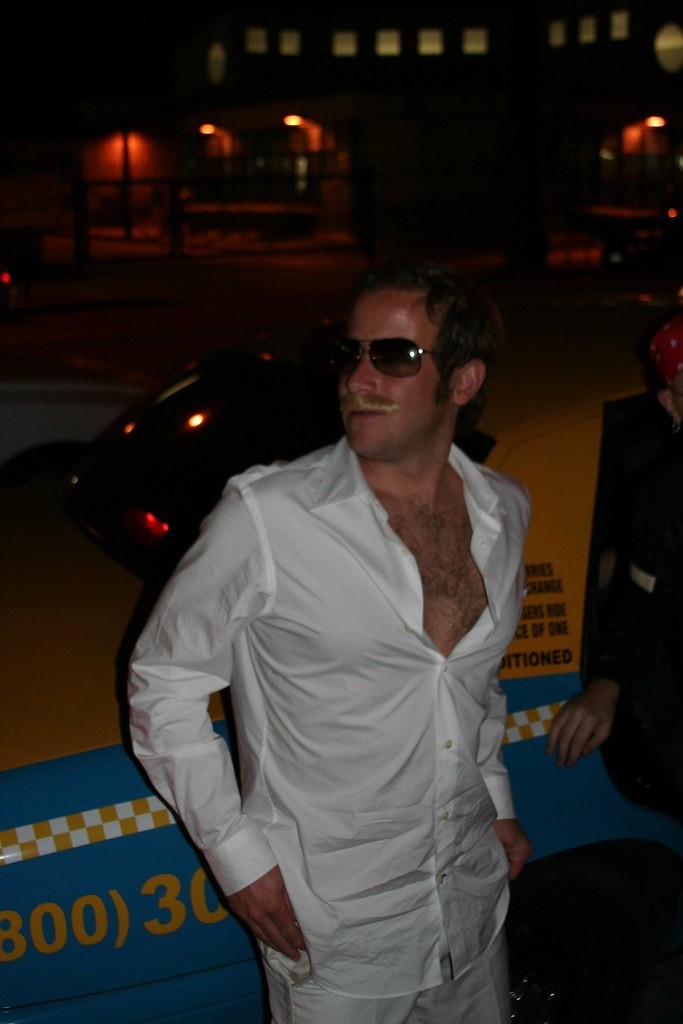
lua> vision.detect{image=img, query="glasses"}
[326,336,446,379]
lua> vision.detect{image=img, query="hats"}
[649,316,683,385]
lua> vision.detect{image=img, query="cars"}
[1,276,682,1023]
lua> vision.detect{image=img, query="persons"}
[545,302,683,823]
[125,259,534,1024]
